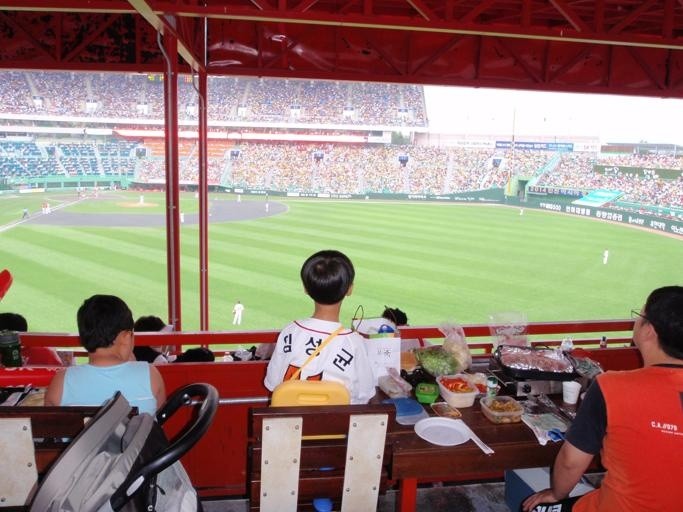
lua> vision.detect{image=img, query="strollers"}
[23,380,219,512]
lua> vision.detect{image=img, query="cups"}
[562,380,583,404]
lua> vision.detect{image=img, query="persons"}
[232,300,245,326]
[180,209,184,223]
[237,194,241,203]
[382,308,429,352]
[44,295,167,424]
[22,207,29,219]
[265,203,269,213]
[602,248,609,265]
[262,250,377,405]
[435,144,683,224]
[0,68,434,196]
[42,199,49,214]
[46,200,51,214]
[139,193,144,205]
[521,285,682,512]
[0,313,64,366]
[133,315,173,365]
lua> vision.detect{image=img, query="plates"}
[415,418,471,446]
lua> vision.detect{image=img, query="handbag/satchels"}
[351,306,401,386]
[270,379,350,440]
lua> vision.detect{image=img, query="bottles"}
[599,336,607,348]
[561,336,573,349]
[1,330,22,367]
[223,352,233,362]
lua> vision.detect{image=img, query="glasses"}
[630,308,649,321]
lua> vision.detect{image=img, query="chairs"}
[244,401,397,512]
[2,397,153,512]
